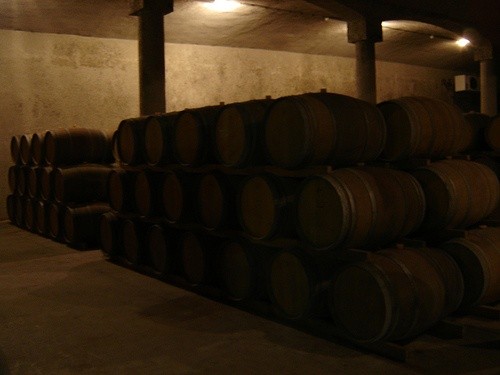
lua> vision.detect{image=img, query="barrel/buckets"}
[6,88,500,348]
